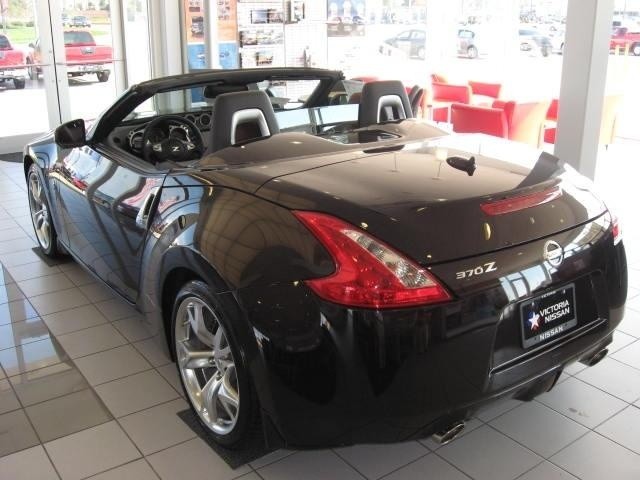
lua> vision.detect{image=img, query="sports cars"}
[22,64,629,453]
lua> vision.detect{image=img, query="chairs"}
[349,74,621,150]
[202,90,278,158]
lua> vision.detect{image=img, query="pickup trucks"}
[0,29,114,89]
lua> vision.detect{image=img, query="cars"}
[60,11,92,29]
[377,23,640,57]
